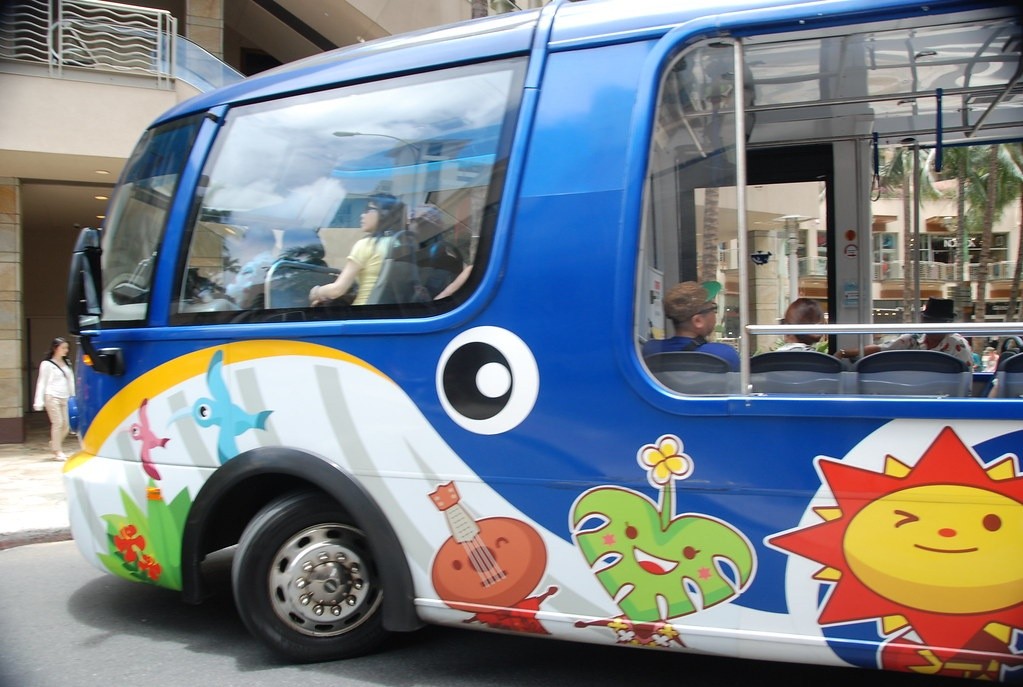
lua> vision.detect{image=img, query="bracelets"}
[840,349,846,357]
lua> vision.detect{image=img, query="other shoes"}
[56,453,67,462]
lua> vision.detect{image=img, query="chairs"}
[644,351,1023,397]
[263,233,463,308]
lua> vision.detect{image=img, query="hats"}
[921,297,958,319]
[664,281,722,318]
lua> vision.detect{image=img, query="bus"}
[55,0,1023,687]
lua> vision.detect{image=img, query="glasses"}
[689,303,718,318]
[365,205,380,211]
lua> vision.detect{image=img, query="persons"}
[194,192,473,312]
[33,337,75,462]
[768,298,825,352]
[640,281,741,372]
[833,297,974,373]
[969,347,1001,398]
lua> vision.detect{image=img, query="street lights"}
[333,127,425,221]
